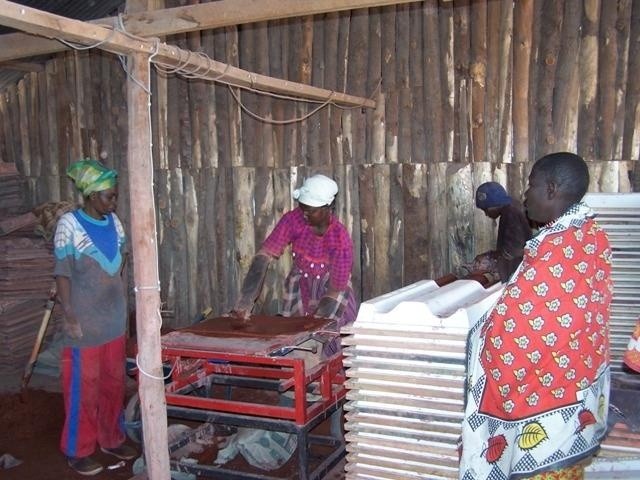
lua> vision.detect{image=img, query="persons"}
[474,181,533,284]
[461,150,615,479]
[56,158,138,475]
[232,173,356,438]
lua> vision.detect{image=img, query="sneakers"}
[68,456,103,475]
[101,444,137,460]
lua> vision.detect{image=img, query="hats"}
[476,182,512,208]
[293,175,338,207]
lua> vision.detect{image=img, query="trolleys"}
[121,312,345,480]
